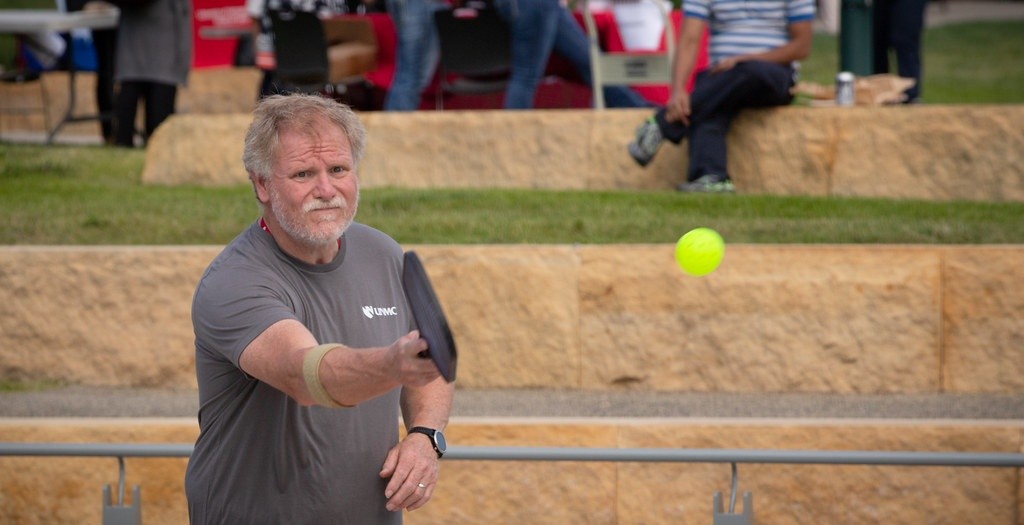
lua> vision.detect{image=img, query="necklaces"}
[260,218,341,251]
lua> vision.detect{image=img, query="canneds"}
[835,72,855,104]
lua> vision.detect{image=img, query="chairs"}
[260,10,331,99]
[432,5,541,112]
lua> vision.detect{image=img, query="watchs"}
[409,427,447,458]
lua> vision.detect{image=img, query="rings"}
[418,484,426,489]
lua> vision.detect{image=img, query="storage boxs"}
[321,17,382,80]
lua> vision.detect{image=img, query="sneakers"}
[677,171,735,192]
[627,115,666,168]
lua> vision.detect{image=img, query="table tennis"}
[675,225,726,277]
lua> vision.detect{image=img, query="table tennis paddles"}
[400,250,460,386]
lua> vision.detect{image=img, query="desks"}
[0,12,125,142]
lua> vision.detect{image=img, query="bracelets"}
[303,343,356,410]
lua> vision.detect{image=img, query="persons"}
[245,0,653,111]
[627,0,817,194]
[66,0,194,148]
[185,91,456,525]
[868,0,929,104]
